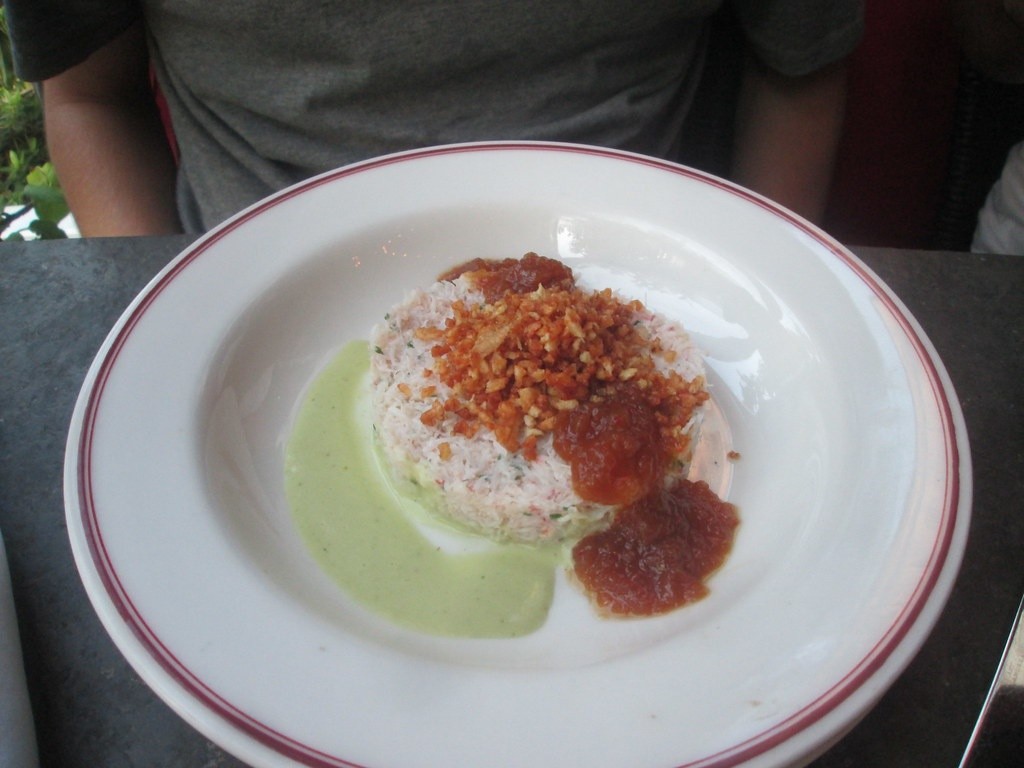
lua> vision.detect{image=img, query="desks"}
[0,236,1024,768]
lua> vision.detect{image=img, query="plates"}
[62,140,971,768]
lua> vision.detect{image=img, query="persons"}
[5,0,870,237]
